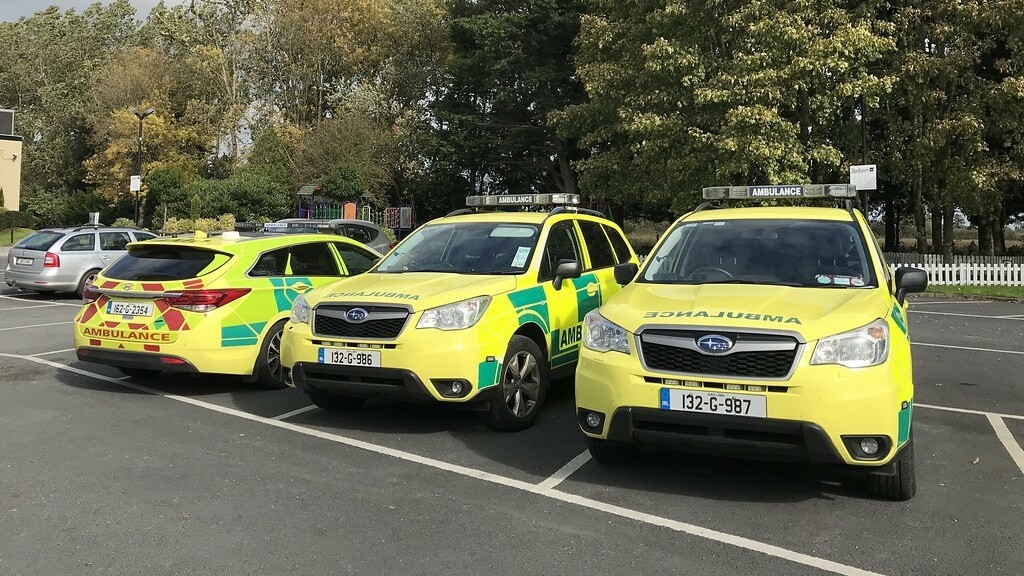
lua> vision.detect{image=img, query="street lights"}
[129,105,156,227]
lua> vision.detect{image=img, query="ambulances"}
[572,185,930,503]
[276,190,641,435]
[73,229,385,388]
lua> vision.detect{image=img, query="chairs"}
[451,234,493,272]
[794,238,859,285]
[690,237,751,280]
[491,235,534,272]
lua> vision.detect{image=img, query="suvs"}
[4,224,160,300]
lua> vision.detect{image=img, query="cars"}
[258,217,395,256]
